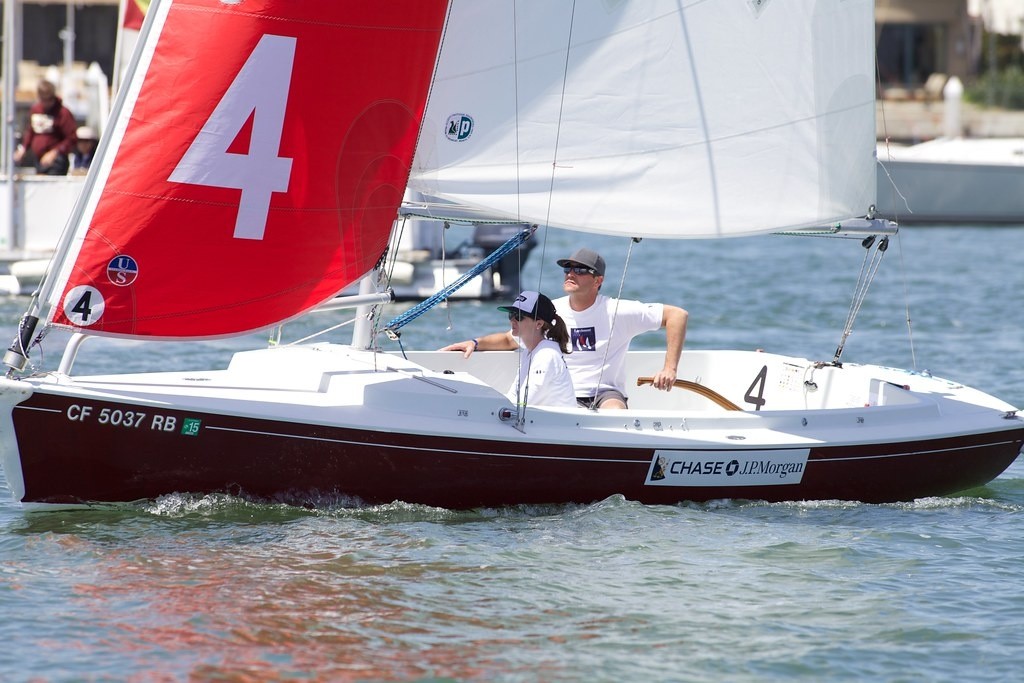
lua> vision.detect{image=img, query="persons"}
[496,291,578,408]
[436,248,688,409]
[14,81,100,175]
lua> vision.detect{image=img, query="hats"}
[557,249,606,276]
[76,127,98,140]
[497,290,555,324]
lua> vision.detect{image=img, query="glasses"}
[508,311,535,321]
[563,265,596,276]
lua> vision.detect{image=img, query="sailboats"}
[0,1,1023,513]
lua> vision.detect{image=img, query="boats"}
[879,133,1024,225]
[338,190,539,295]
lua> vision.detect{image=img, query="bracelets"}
[472,340,478,350]
